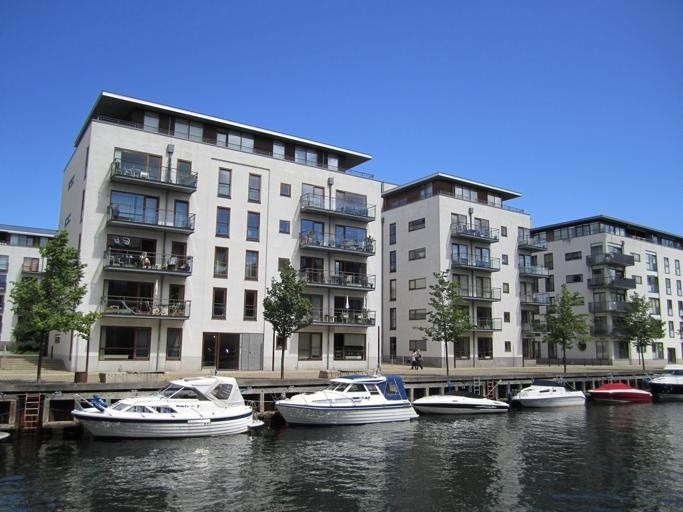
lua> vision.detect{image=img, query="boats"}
[275,373,420,425]
[412,389,510,415]
[512,379,587,408]
[70,375,264,437]
[587,382,652,403]
[651,365,683,399]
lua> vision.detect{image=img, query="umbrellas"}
[345,295,350,323]
[362,297,367,317]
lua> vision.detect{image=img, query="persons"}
[408,349,418,371]
[139,251,150,266]
[415,349,425,371]
